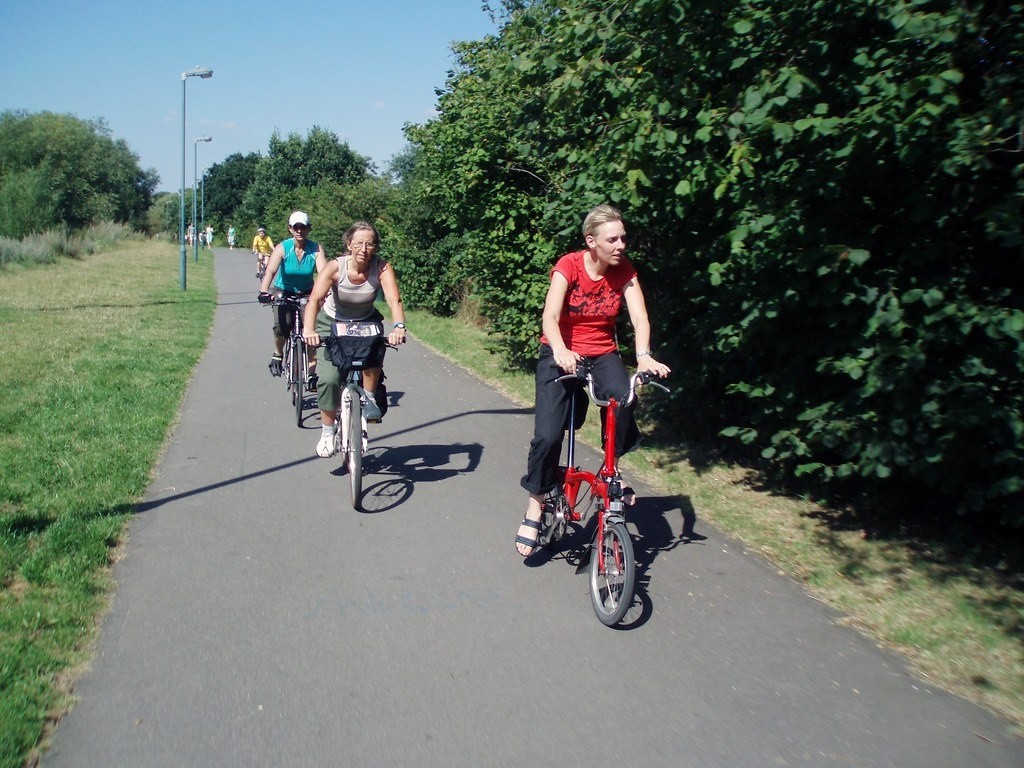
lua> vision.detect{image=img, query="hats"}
[289,212,310,226]
[257,227,266,232]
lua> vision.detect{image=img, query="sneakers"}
[316,433,335,458]
[361,396,385,419]
[309,374,319,392]
[270,352,283,377]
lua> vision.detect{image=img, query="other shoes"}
[256,273,260,278]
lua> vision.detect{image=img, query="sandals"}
[602,474,637,509]
[515,512,543,557]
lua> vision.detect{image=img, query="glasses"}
[351,240,375,251]
[293,224,305,230]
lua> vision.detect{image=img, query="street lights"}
[191,135,213,262]
[178,67,214,294]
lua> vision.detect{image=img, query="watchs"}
[636,351,652,358]
[394,323,405,329]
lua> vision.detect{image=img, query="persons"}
[227,224,236,249]
[515,205,672,556]
[303,221,406,458]
[199,231,206,245]
[188,222,194,247]
[253,228,275,278]
[258,212,330,391]
[175,231,179,243]
[206,224,213,248]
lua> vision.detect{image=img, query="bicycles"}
[259,295,325,428]
[253,252,271,282]
[300,333,407,510]
[534,355,672,628]
